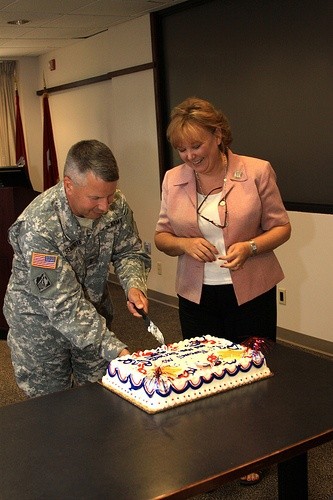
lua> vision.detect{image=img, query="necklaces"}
[195,152,227,205]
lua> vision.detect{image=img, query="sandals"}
[239,466,267,485]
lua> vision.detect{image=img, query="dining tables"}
[0,341,333,500]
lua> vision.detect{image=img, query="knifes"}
[132,302,164,344]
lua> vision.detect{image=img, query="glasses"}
[196,186,228,229]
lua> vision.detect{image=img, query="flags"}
[42,92,59,192]
[14,87,29,173]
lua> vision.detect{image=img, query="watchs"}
[247,240,257,257]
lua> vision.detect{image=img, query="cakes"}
[102,334,270,411]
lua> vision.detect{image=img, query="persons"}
[152,98,292,495]
[2,140,151,397]
